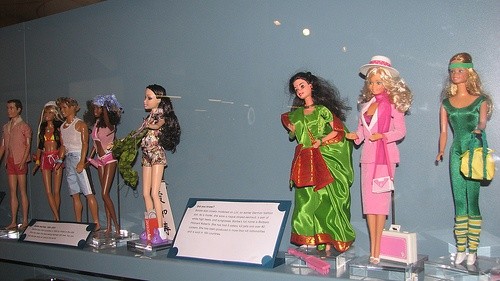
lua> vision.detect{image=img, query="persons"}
[345,56,412,264]
[281,72,356,252]
[55,97,100,232]
[33,101,64,222]
[438,53,491,266]
[83,95,123,234]
[0,99,31,230]
[108,84,180,245]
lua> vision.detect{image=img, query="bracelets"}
[36,160,40,165]
[58,160,62,163]
[320,139,322,145]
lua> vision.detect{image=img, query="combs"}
[288,248,330,276]
